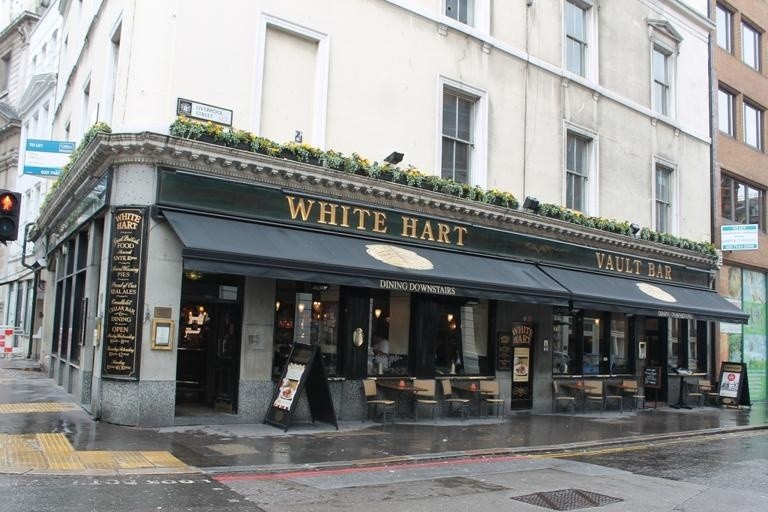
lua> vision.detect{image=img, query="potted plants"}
[168,114,281,155]
[391,166,518,210]
[285,142,389,178]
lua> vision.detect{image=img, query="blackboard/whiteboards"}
[641,367,661,388]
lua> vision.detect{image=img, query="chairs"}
[551,379,639,416]
[362,379,505,427]
[685,380,719,408]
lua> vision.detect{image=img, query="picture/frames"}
[151,318,174,350]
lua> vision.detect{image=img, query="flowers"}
[533,201,631,239]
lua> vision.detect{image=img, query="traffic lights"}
[0,189,23,242]
